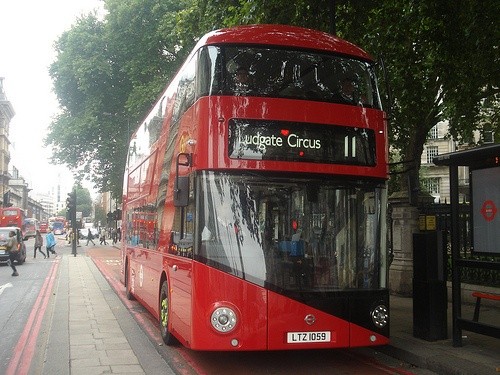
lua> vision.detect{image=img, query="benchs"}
[472,292,500,321]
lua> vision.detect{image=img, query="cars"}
[0,226,27,265]
[39,224,47,234]
[48,216,85,235]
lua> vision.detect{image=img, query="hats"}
[48,229,51,232]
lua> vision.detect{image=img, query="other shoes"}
[44,255,46,259]
[12,272,18,276]
[93,244,95,246]
[52,254,56,256]
[34,256,36,258]
[100,243,102,245]
[104,244,106,245]
[47,257,50,259]
[85,245,88,246]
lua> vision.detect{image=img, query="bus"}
[1,207,26,240]
[118,23,393,351]
[23,218,39,238]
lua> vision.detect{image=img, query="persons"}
[86,228,95,247]
[33,230,47,260]
[45,228,57,258]
[4,232,22,276]
[99,227,122,247]
